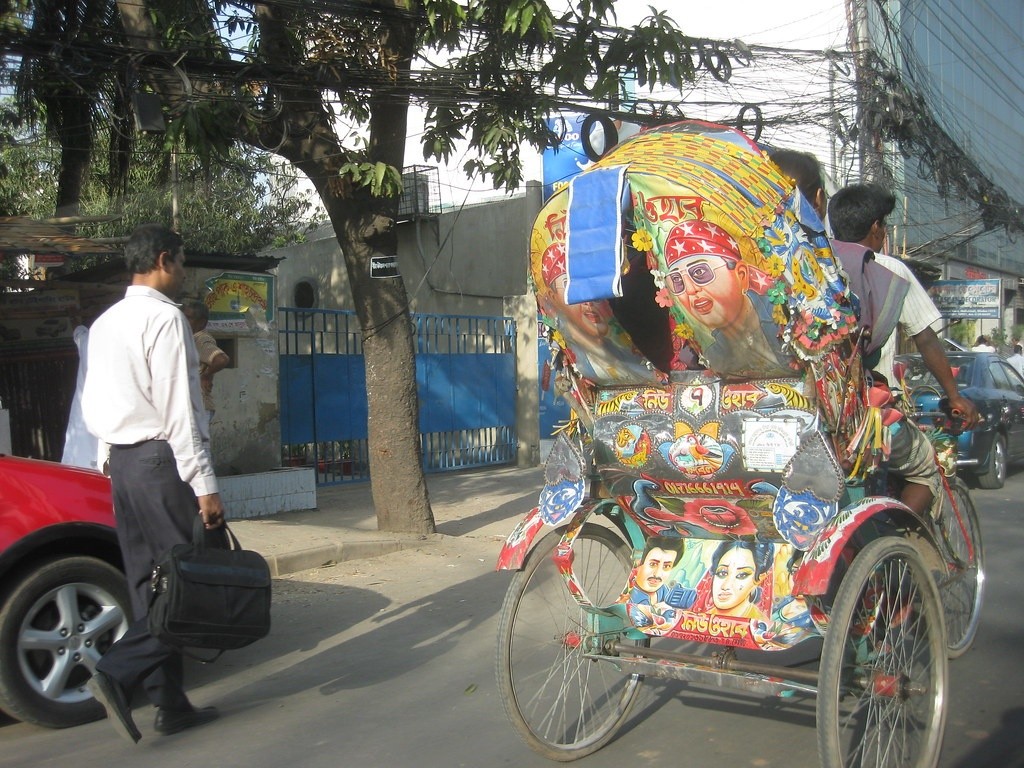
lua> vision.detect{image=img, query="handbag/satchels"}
[133,511,273,664]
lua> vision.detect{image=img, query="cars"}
[0,453,140,729]
[894,350,1024,489]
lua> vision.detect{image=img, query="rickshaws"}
[495,116,987,768]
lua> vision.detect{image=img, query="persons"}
[79,225,226,743]
[772,149,895,385]
[829,181,980,523]
[185,298,231,477]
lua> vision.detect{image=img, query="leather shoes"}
[87,672,143,744]
[152,706,219,735]
